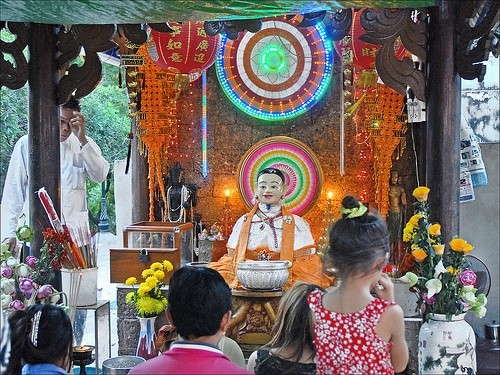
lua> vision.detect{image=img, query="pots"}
[481,320,500,342]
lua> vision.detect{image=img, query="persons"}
[1,91,111,349]
[204,167,323,292]
[1,303,76,375]
[246,280,326,375]
[128,265,258,374]
[382,169,407,261]
[161,161,200,224]
[306,195,410,375]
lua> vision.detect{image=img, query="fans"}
[465,255,491,298]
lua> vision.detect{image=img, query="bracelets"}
[80,139,89,148]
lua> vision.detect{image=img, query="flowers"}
[399,186,487,321]
[125,260,174,318]
[0,221,69,310]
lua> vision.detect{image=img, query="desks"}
[224,290,282,345]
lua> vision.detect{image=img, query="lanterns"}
[330,8,411,84]
[144,17,225,90]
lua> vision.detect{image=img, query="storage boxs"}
[71,300,111,375]
[123,221,194,269]
[109,247,180,285]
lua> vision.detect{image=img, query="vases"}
[135,315,159,360]
[417,313,477,375]
